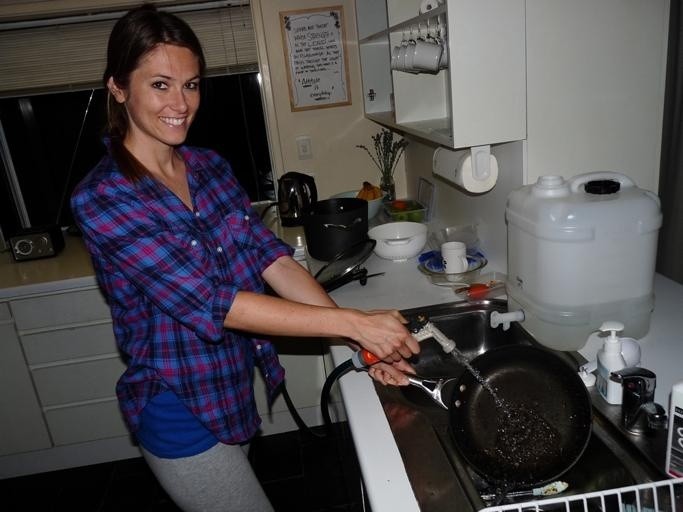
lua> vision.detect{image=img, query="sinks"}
[375,299,678,511]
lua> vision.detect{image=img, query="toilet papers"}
[429,145,499,195]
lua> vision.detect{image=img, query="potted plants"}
[356,129,410,201]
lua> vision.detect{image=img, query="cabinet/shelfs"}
[0,275,128,481]
[355,0,527,149]
[253,259,346,436]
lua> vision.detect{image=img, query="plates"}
[420,0,446,15]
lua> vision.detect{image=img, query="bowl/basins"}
[417,252,489,288]
[367,222,428,262]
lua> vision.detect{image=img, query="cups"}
[391,32,449,74]
[440,241,470,281]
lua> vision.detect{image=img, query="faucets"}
[490,308,526,333]
[611,366,668,439]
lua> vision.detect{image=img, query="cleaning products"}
[594,320,627,405]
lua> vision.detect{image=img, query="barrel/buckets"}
[505,170,664,352]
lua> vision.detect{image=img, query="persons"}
[72,8,419,512]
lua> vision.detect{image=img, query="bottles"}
[665,371,683,485]
[276,172,317,228]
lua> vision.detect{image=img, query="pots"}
[302,197,368,263]
[361,343,594,486]
